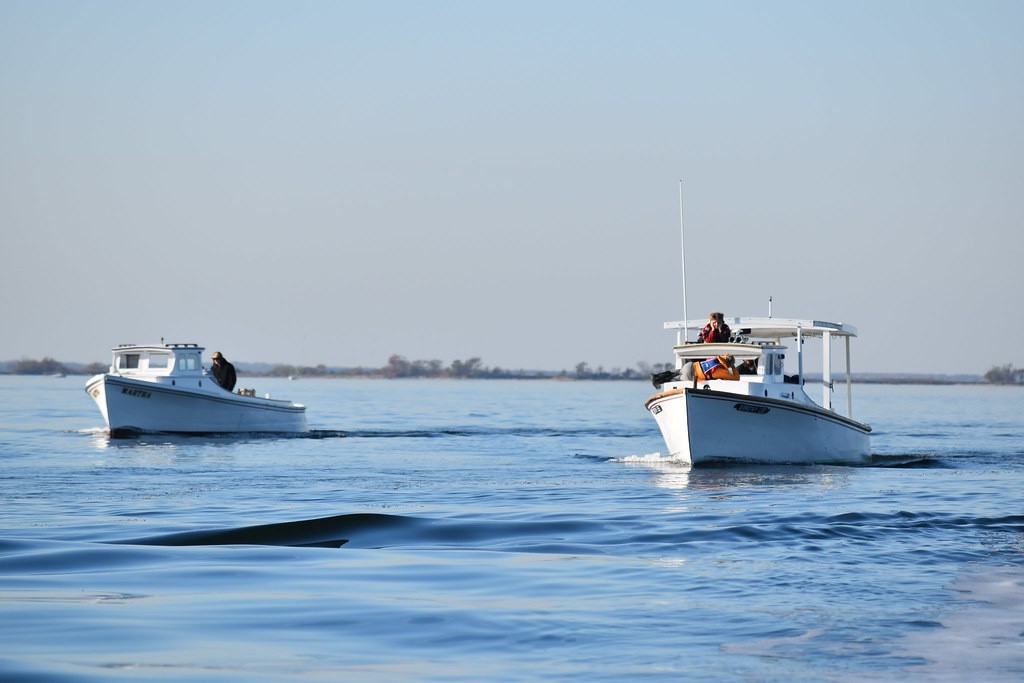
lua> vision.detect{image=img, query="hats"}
[717,354,735,368]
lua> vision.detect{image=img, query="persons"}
[737,360,757,374]
[211,351,236,391]
[650,353,740,390]
[702,312,731,343]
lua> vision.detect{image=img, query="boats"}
[645,181,875,466]
[86,337,311,437]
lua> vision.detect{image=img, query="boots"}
[653,376,672,390]
[650,370,672,382]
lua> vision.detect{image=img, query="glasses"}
[212,358,220,360]
